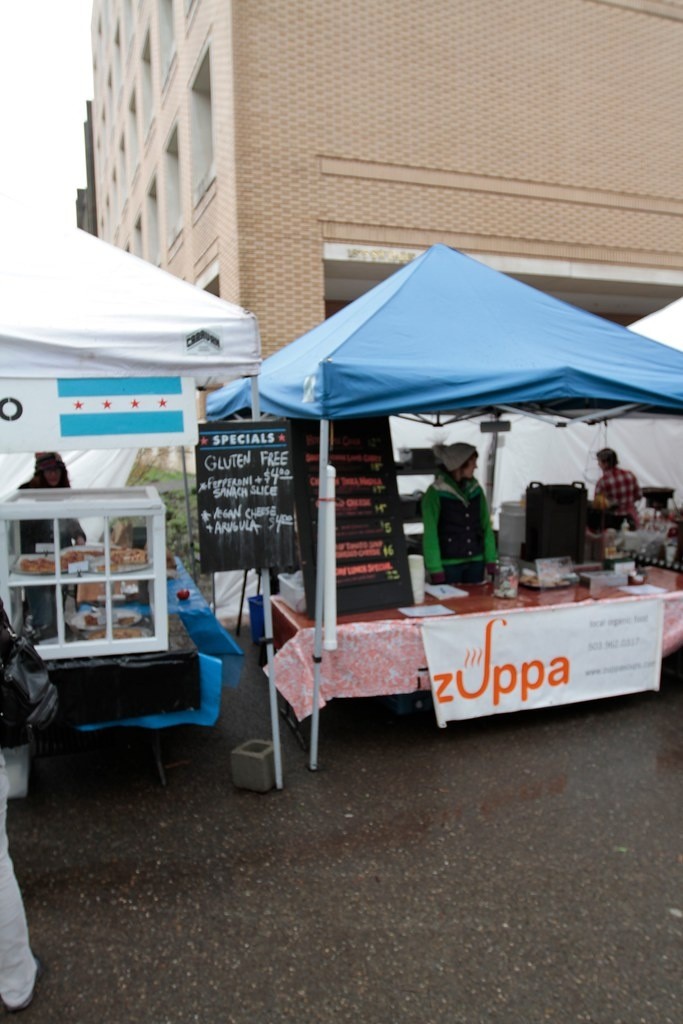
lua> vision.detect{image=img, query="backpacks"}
[0,599,57,749]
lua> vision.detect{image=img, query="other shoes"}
[7,955,42,1011]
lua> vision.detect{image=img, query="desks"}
[269,568,683,772]
[48,556,244,787]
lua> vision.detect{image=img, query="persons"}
[596,448,643,529]
[14,450,87,631]
[421,441,499,584]
[0,752,43,1011]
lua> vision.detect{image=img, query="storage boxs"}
[248,594,265,645]
[604,560,635,572]
[579,571,628,588]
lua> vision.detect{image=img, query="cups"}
[407,554,425,605]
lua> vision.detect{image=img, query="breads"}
[85,614,142,639]
[20,548,147,572]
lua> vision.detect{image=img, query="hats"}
[35,452,65,474]
[433,443,476,471]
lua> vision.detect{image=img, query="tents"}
[0,219,286,791]
[203,244,683,772]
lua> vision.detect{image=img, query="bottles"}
[492,553,519,599]
[498,500,526,555]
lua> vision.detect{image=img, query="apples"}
[177,589,189,600]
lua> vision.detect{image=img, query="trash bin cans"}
[248,595,264,643]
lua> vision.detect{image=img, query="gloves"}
[486,563,497,574]
[431,573,445,584]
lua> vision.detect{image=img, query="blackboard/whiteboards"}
[194,420,303,574]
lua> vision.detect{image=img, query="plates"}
[71,608,143,631]
[90,557,152,573]
[61,545,104,555]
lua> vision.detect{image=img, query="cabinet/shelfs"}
[0,487,170,663]
[396,462,447,551]
